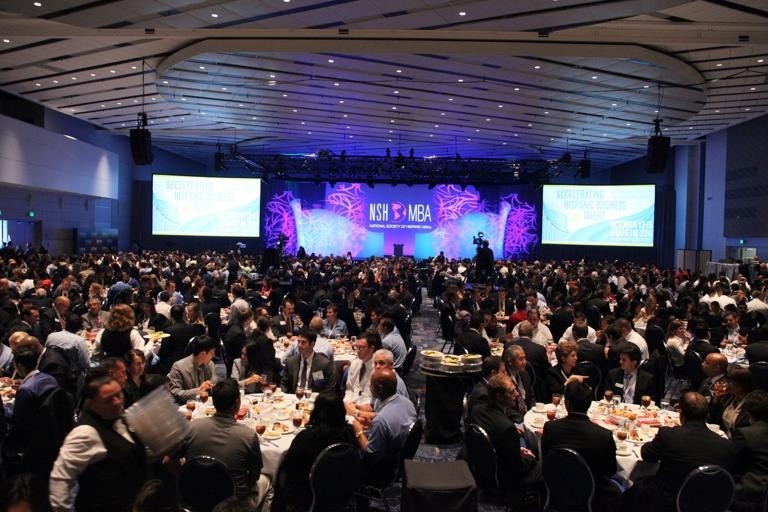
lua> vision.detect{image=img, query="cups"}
[534,418,544,425]
[535,402,544,411]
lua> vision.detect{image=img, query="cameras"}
[278,232,290,244]
[473,231,484,246]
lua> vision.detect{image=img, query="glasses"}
[354,345,369,350]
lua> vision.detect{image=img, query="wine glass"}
[182,410,191,422]
[199,391,208,408]
[286,332,291,341]
[604,391,613,406]
[283,340,289,352]
[303,389,311,402]
[269,382,277,396]
[292,413,301,434]
[551,393,561,409]
[546,409,555,421]
[641,395,650,413]
[185,399,195,412]
[254,423,265,444]
[616,429,626,450]
[295,386,304,405]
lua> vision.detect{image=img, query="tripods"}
[462,247,482,285]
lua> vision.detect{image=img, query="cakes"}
[303,408,311,419]
[237,407,247,419]
[296,401,310,410]
[272,421,289,432]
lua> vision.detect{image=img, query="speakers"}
[215,152,225,171]
[581,160,592,178]
[647,134,670,174]
[130,128,154,166]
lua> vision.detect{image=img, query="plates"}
[531,407,547,413]
[529,422,544,429]
[266,399,294,439]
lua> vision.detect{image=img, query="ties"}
[61,314,65,329]
[624,375,631,403]
[95,315,100,328]
[299,361,307,389]
[501,301,504,312]
[286,317,291,332]
[358,363,364,382]
[474,302,478,311]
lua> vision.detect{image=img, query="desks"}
[706,260,740,281]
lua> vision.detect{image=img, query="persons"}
[2,235,767,510]
